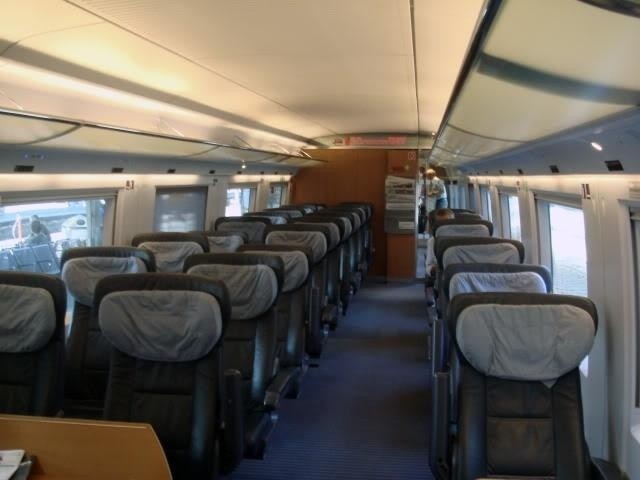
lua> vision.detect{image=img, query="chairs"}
[132,232,209,271]
[428,263,552,327]
[428,209,493,238]
[426,237,525,306]
[60,247,149,413]
[0,269,67,416]
[214,213,266,245]
[245,203,373,409]
[431,292,631,480]
[95,271,227,480]
[203,228,247,255]
[183,253,295,458]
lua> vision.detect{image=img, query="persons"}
[425,168,448,208]
[417,166,426,234]
[425,211,455,289]
